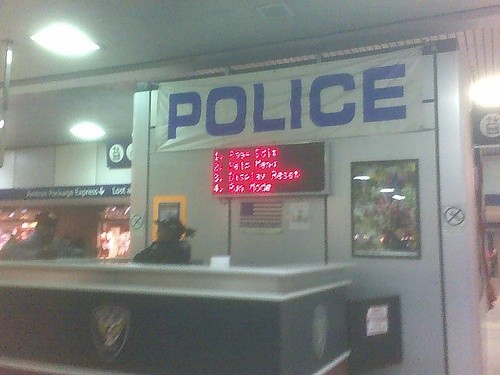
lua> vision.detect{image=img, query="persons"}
[130,217,196,265]
[13,208,72,261]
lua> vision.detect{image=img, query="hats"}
[153,217,186,233]
